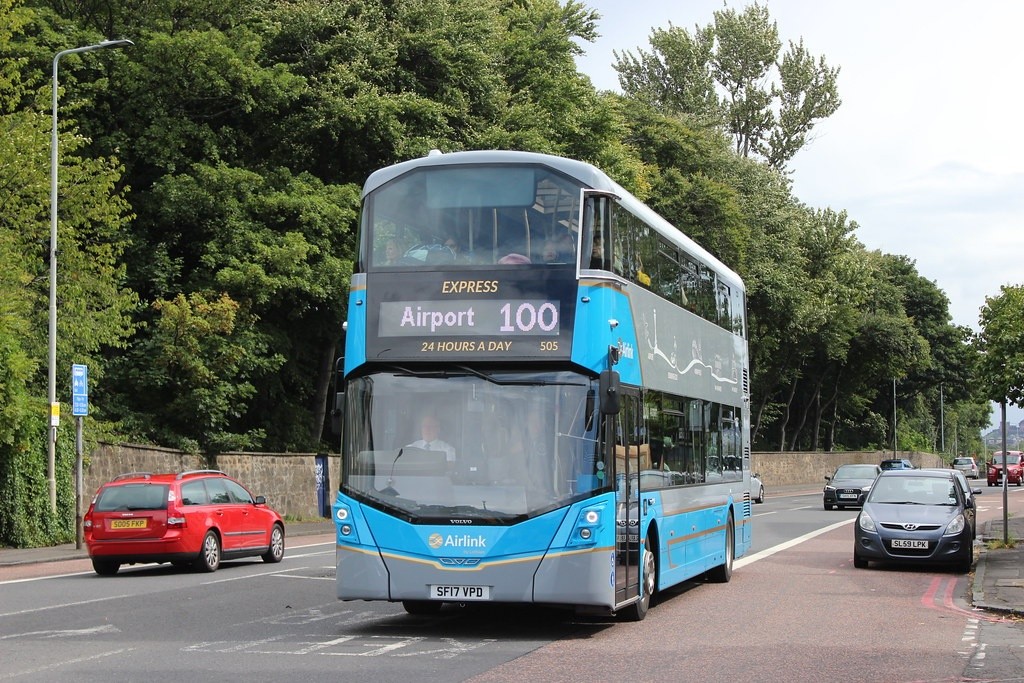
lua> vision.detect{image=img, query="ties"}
[424,444,430,452]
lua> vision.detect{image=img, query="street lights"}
[47,38,136,512]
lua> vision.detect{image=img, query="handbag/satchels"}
[402,244,456,265]
[498,253,531,264]
[637,270,651,287]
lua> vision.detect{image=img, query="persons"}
[385,235,650,286]
[402,417,456,476]
[481,404,552,488]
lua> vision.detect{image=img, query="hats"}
[593,231,605,238]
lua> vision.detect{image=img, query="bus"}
[332,148,752,625]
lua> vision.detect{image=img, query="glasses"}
[444,243,457,249]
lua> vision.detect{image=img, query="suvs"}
[82,469,287,575]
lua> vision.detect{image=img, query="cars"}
[751,471,766,505]
[604,460,742,565]
[918,466,982,539]
[823,463,881,512]
[985,451,1024,488]
[953,457,980,480]
[879,457,915,472]
[853,469,976,572]
